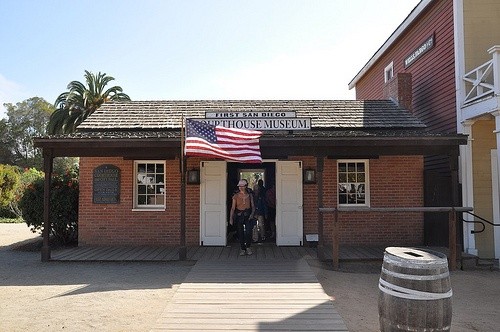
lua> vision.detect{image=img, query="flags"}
[184,117,263,164]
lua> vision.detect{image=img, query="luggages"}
[252,215,264,243]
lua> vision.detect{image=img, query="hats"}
[237,179,247,187]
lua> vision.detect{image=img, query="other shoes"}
[246,248,252,255]
[240,250,246,255]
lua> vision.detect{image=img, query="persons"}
[258,179,267,194]
[268,183,276,238]
[229,179,255,256]
[234,179,252,194]
[251,185,269,242]
